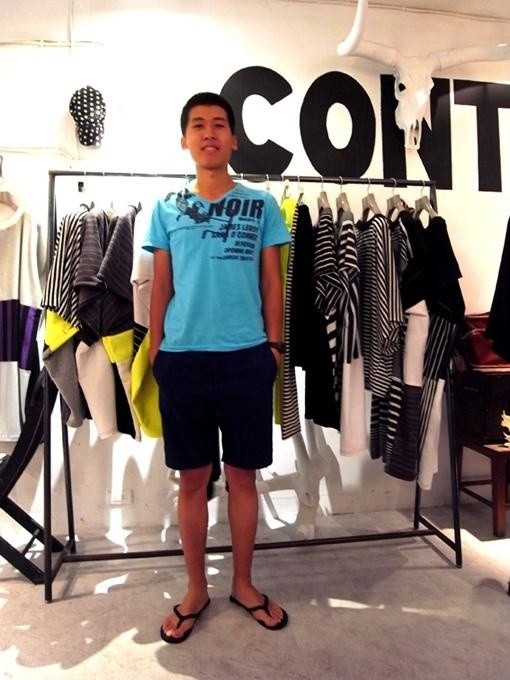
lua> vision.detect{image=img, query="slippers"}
[161,596,209,643]
[230,593,288,631]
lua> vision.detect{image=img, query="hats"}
[69,86,105,145]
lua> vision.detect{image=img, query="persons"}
[138,89,304,646]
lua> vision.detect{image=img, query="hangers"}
[0,157,436,230]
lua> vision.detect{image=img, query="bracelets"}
[268,341,285,355]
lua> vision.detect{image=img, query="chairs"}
[450,311,510,537]
